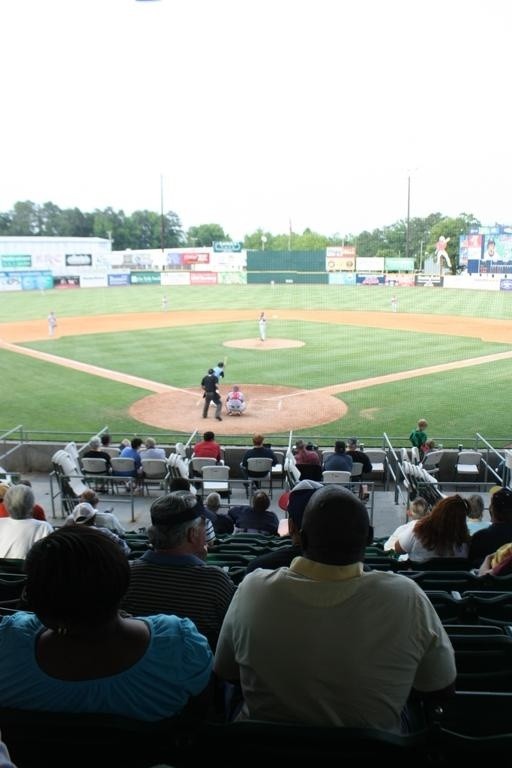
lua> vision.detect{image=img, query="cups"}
[438,444,442,451]
[359,444,363,451]
[362,485,367,493]
[458,445,463,451]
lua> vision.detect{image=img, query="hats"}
[348,437,360,445]
[152,494,218,525]
[287,478,326,528]
[71,501,99,526]
[208,369,215,374]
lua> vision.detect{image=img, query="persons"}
[295,436,372,497]
[0,733,16,767]
[204,479,322,574]
[213,481,460,735]
[0,473,54,560]
[213,361,225,386]
[0,521,216,720]
[243,434,278,487]
[162,295,168,310]
[119,489,236,652]
[392,294,398,312]
[193,432,222,478]
[409,420,435,461]
[83,433,166,491]
[48,310,57,337]
[225,385,248,415]
[202,368,223,421]
[385,481,512,576]
[259,312,267,339]
[65,488,130,554]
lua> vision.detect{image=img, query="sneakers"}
[215,416,222,421]
[95,486,138,494]
